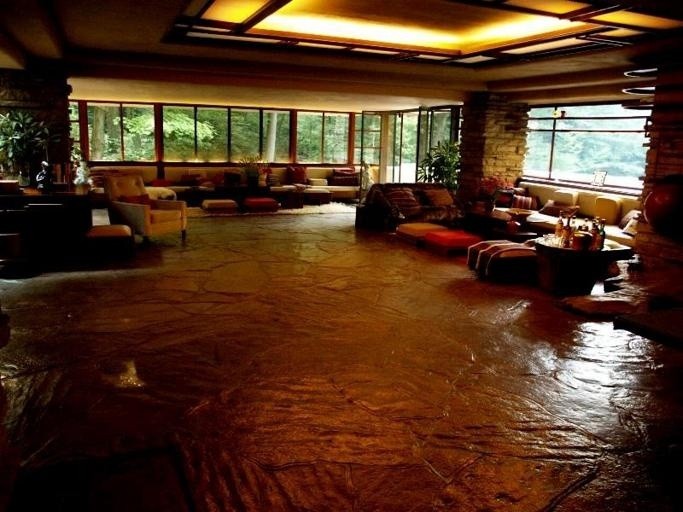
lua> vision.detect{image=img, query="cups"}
[542,233,560,247]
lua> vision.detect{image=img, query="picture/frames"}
[589,170,607,189]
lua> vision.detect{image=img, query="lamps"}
[551,109,566,118]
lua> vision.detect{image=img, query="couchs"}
[83,161,379,213]
[0,160,136,275]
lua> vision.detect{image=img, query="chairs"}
[104,169,187,245]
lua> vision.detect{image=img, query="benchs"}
[356,177,644,298]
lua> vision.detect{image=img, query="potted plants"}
[0,106,50,186]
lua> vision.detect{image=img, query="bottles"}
[556,209,606,250]
